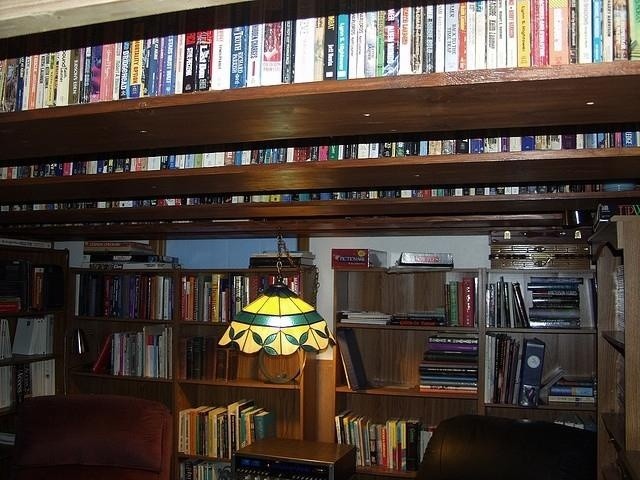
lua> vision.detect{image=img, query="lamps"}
[213,229,337,361]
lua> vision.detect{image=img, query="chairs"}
[9,392,175,479]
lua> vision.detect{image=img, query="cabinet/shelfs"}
[327,211,640,478]
[59,264,318,479]
[0,314,57,369]
[229,436,358,480]
[0,0,639,237]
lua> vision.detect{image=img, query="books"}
[2,240,55,446]
[1,0,639,224]
[418,333,478,394]
[177,273,302,321]
[484,333,596,406]
[334,411,437,473]
[249,251,315,267]
[177,398,276,480]
[72,273,174,380]
[485,277,583,329]
[338,276,478,326]
[79,242,179,269]
[178,335,239,383]
[337,329,366,390]
